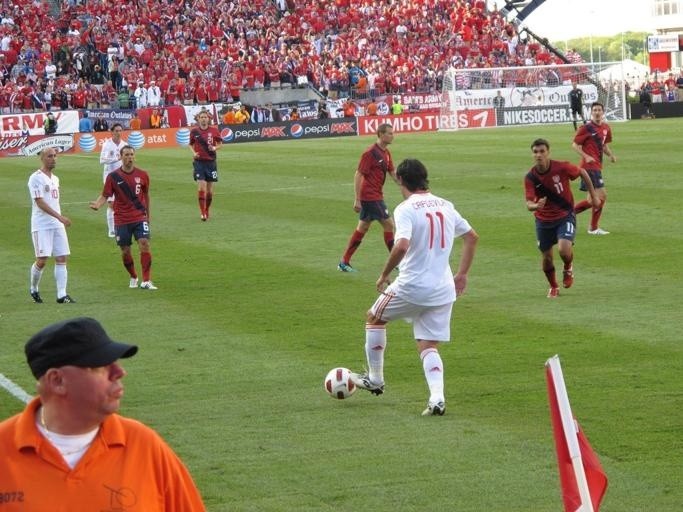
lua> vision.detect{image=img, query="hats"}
[25,317,139,380]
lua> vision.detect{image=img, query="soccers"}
[325,367,356,399]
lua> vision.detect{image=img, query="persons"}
[189,111,223,221]
[27,146,77,303]
[572,102,615,234]
[337,124,401,273]
[350,159,479,416]
[524,138,602,297]
[2,1,680,137]
[99,123,128,238]
[89,145,158,290]
[1,317,205,512]
[567,81,586,131]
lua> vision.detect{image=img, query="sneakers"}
[587,228,610,235]
[57,295,78,303]
[350,373,385,396]
[108,231,115,237]
[421,402,445,415]
[141,280,158,290]
[337,262,355,272]
[563,268,572,289]
[128,277,139,288]
[547,285,559,297]
[30,289,43,303]
[201,213,207,221]
